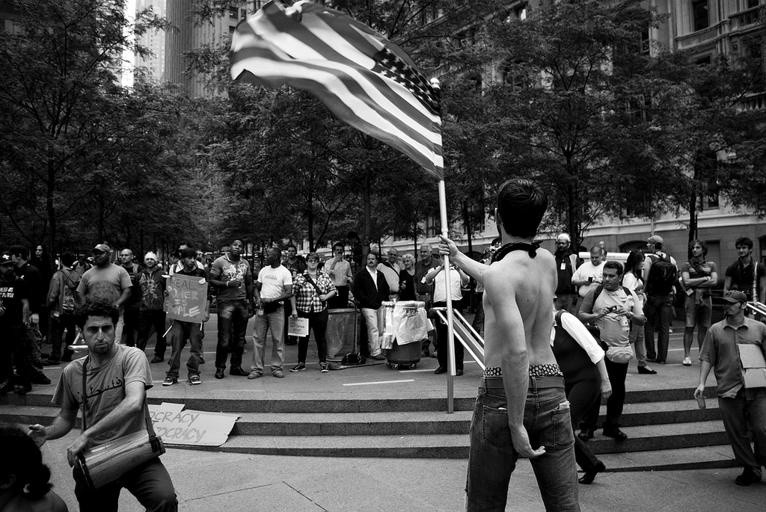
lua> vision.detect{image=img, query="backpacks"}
[649,251,677,296]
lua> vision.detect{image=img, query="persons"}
[576,262,648,442]
[26,303,178,512]
[547,306,616,484]
[438,180,581,512]
[0,425,68,512]
[692,289,766,486]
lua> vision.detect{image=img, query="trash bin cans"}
[325,308,362,366]
[382,300,426,370]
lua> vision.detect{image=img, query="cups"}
[697,395,706,409]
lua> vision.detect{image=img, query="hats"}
[179,249,198,259]
[722,290,748,306]
[648,234,665,244]
[92,243,110,254]
[1,253,13,265]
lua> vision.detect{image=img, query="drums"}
[76,428,166,488]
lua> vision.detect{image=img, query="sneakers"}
[14,385,33,396]
[188,375,202,386]
[602,426,628,442]
[577,427,594,439]
[369,353,387,361]
[638,363,658,378]
[656,355,666,364]
[456,368,464,377]
[578,462,607,485]
[734,469,762,485]
[272,370,284,378]
[320,361,329,374]
[229,367,249,376]
[683,356,695,368]
[163,374,178,387]
[646,355,656,362]
[289,363,307,373]
[248,369,264,379]
[214,368,225,379]
[151,355,164,365]
[434,365,448,374]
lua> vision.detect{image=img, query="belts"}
[479,374,564,392]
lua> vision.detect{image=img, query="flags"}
[229,1,446,183]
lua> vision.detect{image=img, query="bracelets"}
[627,311,634,319]
